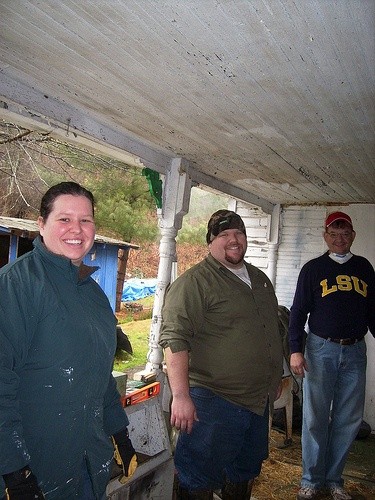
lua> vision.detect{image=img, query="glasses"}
[326,231,352,238]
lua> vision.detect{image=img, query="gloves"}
[2,465,45,500]
[112,428,138,484]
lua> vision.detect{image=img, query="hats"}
[326,212,354,229]
[206,210,246,245]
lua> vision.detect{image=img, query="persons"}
[158,209,284,500]
[285,211,375,500]
[0,181,138,500]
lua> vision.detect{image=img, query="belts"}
[323,336,364,345]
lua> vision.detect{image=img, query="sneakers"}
[296,488,320,500]
[330,487,352,500]
